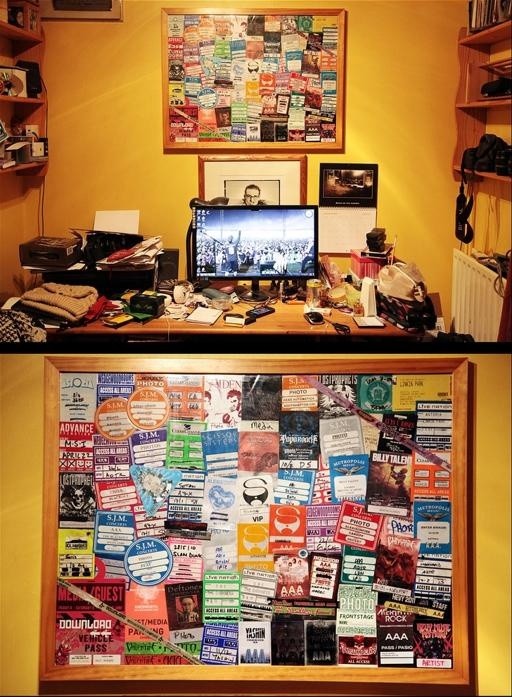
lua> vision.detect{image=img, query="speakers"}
[15,60,42,98]
[157,248,179,281]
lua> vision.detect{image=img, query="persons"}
[389,464,410,500]
[242,185,262,205]
[278,557,304,585]
[176,595,199,622]
[195,226,315,275]
[202,388,242,430]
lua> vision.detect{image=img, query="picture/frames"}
[37,354,472,687]
[160,7,347,152]
[197,153,307,207]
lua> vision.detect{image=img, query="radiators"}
[450,247,507,342]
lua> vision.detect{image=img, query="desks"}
[47,285,427,353]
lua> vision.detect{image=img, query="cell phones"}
[246,305,275,317]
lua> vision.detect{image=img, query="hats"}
[18,281,100,323]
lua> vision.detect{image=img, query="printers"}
[42,210,157,300]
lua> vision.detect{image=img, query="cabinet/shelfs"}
[0,20,49,176]
[451,20,512,185]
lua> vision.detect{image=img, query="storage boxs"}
[42,259,159,300]
[350,248,387,279]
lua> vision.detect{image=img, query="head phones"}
[158,280,194,305]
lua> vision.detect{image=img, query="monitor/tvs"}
[191,204,319,301]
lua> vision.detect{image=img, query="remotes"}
[202,288,231,300]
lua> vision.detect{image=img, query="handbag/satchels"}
[374,261,437,334]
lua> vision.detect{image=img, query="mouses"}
[304,311,325,325]
